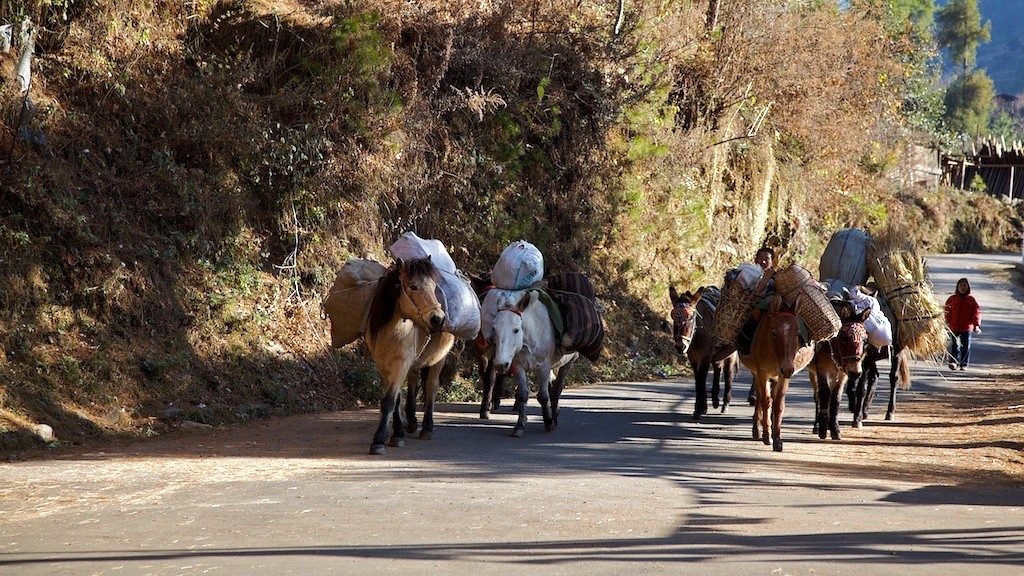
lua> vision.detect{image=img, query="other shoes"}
[961,366,968,371]
[949,363,958,370]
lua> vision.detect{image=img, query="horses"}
[670,285,739,417]
[470,285,520,418]
[736,291,815,452]
[363,254,463,454]
[493,287,578,434]
[807,294,867,440]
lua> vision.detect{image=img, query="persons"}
[747,247,775,404]
[944,278,981,371]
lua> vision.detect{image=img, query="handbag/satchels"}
[709,264,893,355]
